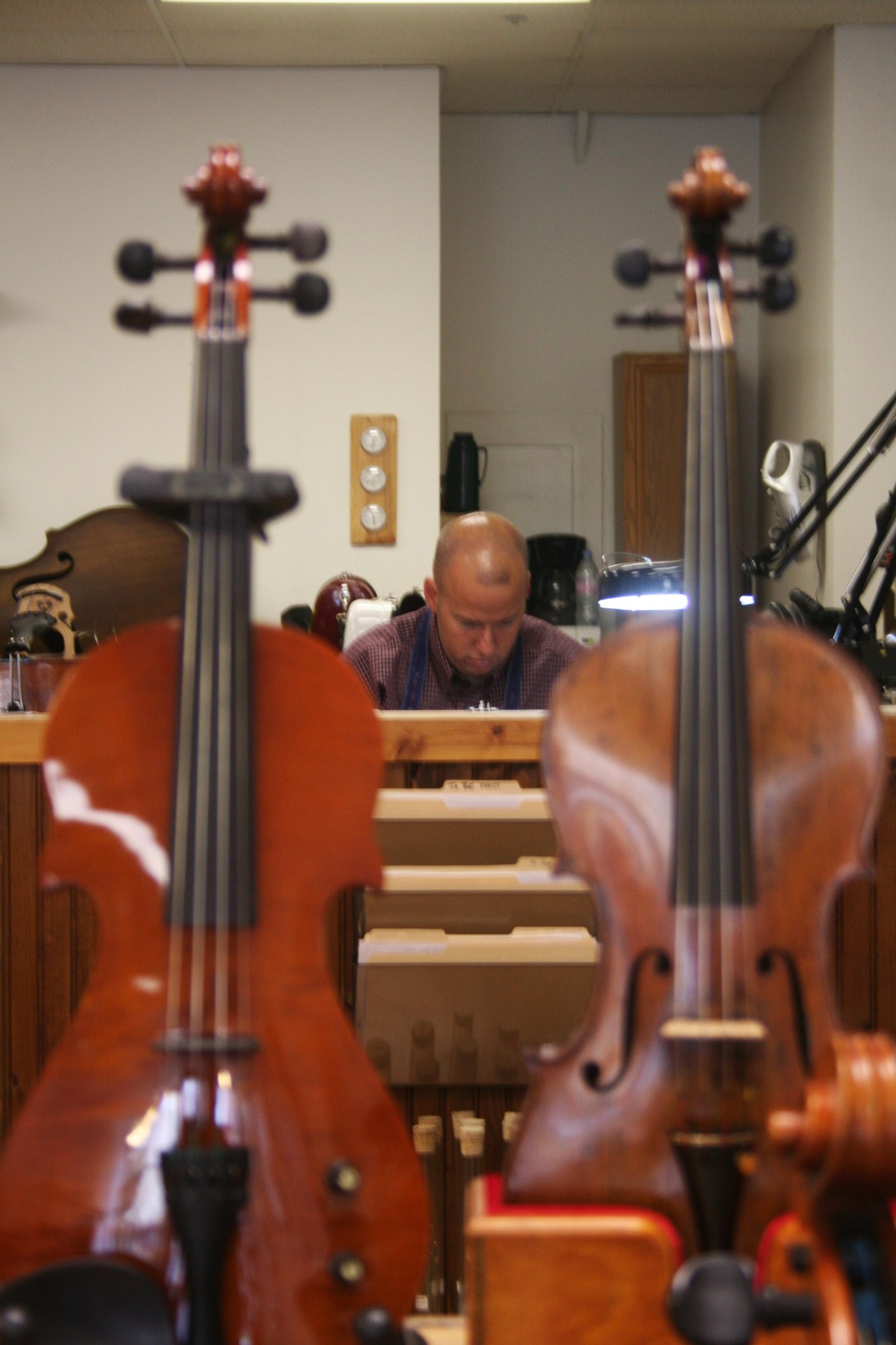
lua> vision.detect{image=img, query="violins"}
[0,136,438,1344]
[500,147,887,1255]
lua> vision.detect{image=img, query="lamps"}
[595,557,688,614]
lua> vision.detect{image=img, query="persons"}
[342,510,595,789]
